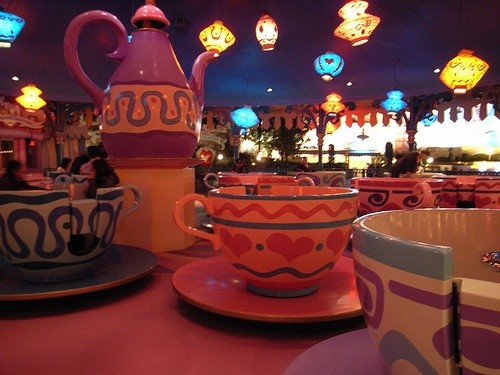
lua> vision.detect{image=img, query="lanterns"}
[255,14,279,50]
[198,20,236,58]
[333,1,381,47]
[420,112,437,126]
[15,86,47,113]
[438,48,490,94]
[326,118,341,134]
[0,10,26,48]
[230,108,260,134]
[321,93,346,116]
[313,53,345,81]
[380,91,409,116]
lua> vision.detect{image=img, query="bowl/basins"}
[352,208,499,375]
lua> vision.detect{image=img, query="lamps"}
[380,59,408,115]
[254,11,279,52]
[15,82,47,113]
[199,20,237,58]
[0,7,26,49]
[333,0,381,46]
[229,104,260,128]
[314,51,345,80]
[438,0,490,95]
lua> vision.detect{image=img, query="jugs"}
[64,0,219,159]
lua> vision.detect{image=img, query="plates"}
[0,244,159,300]
[171,253,364,322]
[284,328,382,375]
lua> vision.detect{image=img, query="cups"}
[55,174,88,185]
[0,185,144,285]
[171,185,359,298]
[204,171,500,216]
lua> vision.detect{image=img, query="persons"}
[390,150,430,178]
[84,159,120,200]
[57,157,71,172]
[327,144,336,163]
[70,142,108,175]
[0,160,46,191]
[232,158,249,174]
[382,142,393,171]
